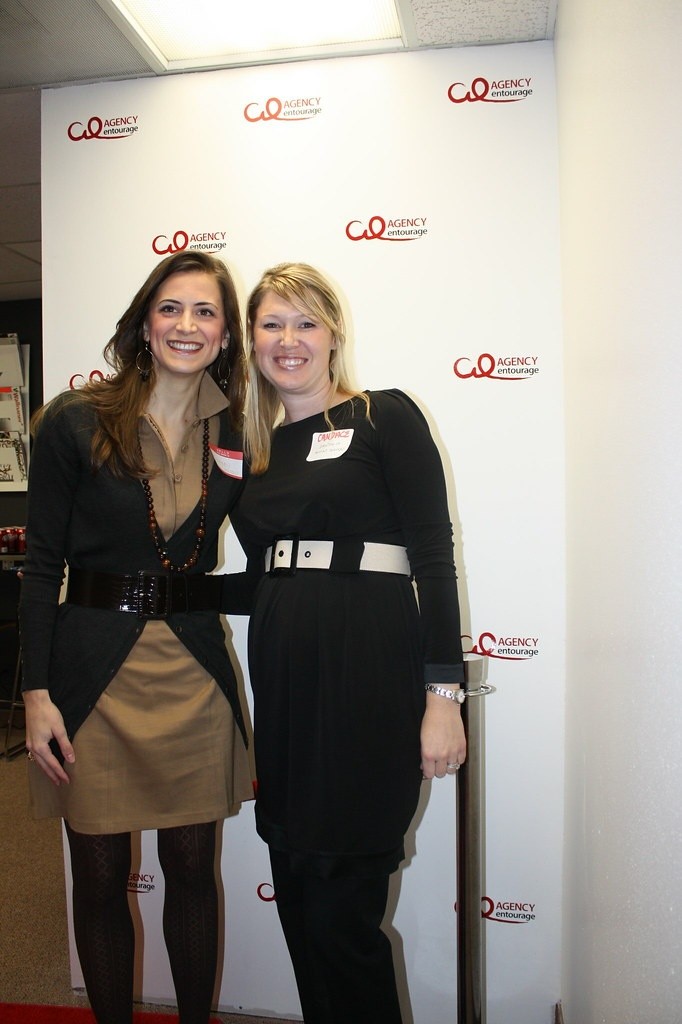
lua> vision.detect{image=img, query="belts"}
[66,570,218,619]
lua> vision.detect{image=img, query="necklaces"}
[131,418,212,574]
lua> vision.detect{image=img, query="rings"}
[446,761,461,770]
[26,751,36,762]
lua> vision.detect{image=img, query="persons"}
[19,250,254,1024]
[202,261,468,1024]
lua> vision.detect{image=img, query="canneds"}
[0,527,26,554]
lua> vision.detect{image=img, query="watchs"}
[424,683,465,706]
